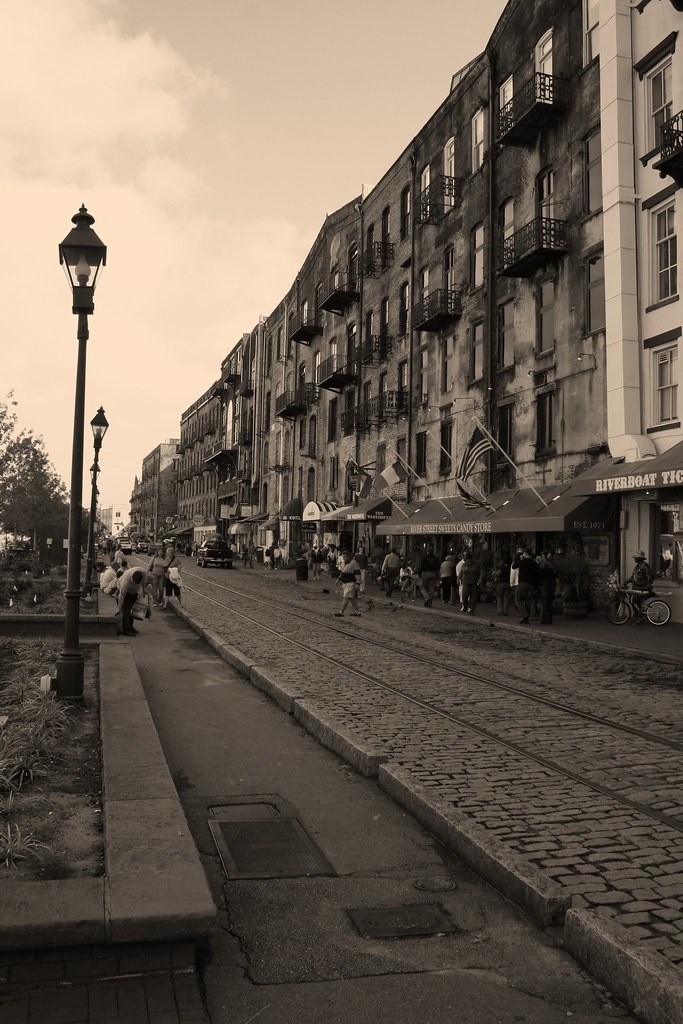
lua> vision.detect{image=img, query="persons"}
[99,538,655,637]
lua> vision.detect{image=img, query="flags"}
[372,460,410,490]
[347,461,371,498]
[455,427,494,482]
[456,483,491,510]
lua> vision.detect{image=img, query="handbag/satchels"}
[114,610,124,633]
[130,592,154,621]
[162,577,170,587]
[461,563,474,584]
[402,576,411,581]
[148,556,155,571]
[145,575,152,589]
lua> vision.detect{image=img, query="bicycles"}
[605,583,672,627]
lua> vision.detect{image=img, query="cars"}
[196,540,234,569]
[116,535,165,557]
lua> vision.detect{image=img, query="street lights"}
[51,203,108,710]
[78,405,110,601]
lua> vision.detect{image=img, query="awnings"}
[167,514,277,534]
[376,483,592,534]
[571,441,683,495]
[279,497,393,519]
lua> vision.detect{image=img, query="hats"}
[112,563,120,570]
[116,571,124,578]
[632,550,647,559]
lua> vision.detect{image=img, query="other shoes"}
[161,606,168,610]
[498,611,553,625]
[152,603,162,607]
[424,597,432,607]
[385,592,392,597]
[334,612,344,617]
[467,608,474,615]
[124,628,138,637]
[179,602,183,607]
[350,611,361,616]
[460,606,467,613]
